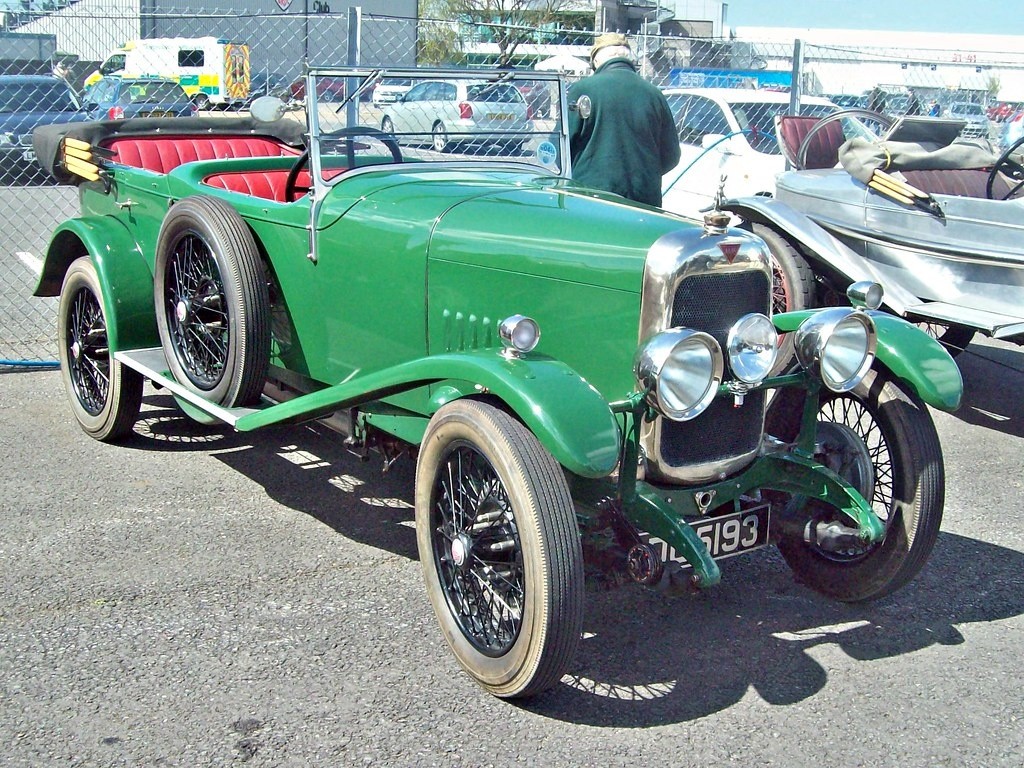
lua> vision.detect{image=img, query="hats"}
[591,33,630,71]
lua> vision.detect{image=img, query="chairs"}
[146,88,160,98]
[775,115,845,171]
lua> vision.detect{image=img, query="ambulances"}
[84,35,250,111]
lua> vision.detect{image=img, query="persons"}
[860,82,943,144]
[490,57,518,101]
[52,56,83,108]
[547,31,682,205]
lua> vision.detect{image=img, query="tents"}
[532,50,597,88]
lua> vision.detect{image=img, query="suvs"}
[699,113,1024,359]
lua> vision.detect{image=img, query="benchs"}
[169,154,424,203]
[886,169,1024,200]
[98,135,304,174]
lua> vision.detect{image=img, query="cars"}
[372,78,414,108]
[83,75,199,121]
[31,64,964,700]
[0,73,100,184]
[661,86,883,227]
[511,80,551,120]
[379,79,534,153]
[947,101,990,139]
[250,72,383,102]
[817,92,928,116]
